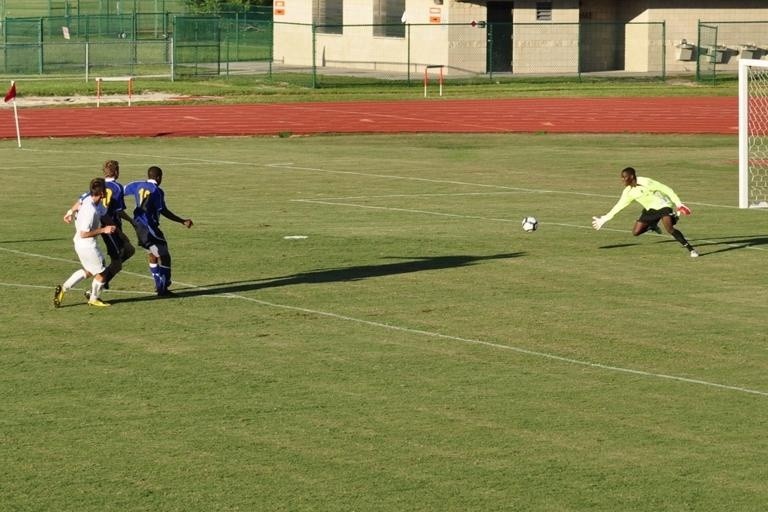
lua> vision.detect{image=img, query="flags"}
[4,80,16,103]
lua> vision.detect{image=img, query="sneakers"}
[54,284,63,308]
[690,249,699,258]
[652,224,661,234]
[84,289,111,307]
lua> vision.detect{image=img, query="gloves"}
[677,204,691,217]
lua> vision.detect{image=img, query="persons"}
[122,166,195,302]
[53,177,112,309]
[61,159,136,301]
[590,165,699,261]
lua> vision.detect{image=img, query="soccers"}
[520,216,538,233]
